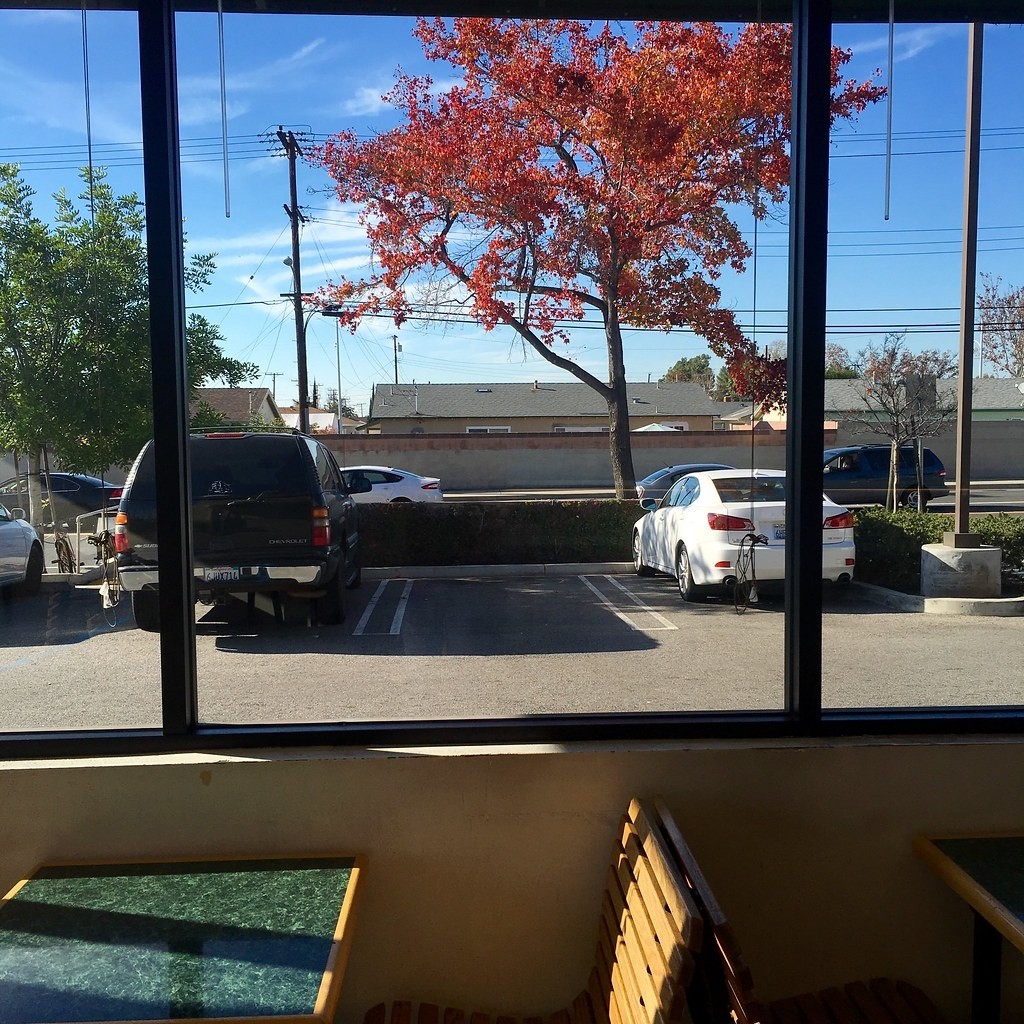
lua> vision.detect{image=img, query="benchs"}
[369,797,761,1024]
[648,795,952,1024]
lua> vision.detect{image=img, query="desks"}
[0,836,365,1024]
[912,833,1024,1024]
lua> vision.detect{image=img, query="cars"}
[0,469,126,533]
[633,460,743,496]
[627,465,863,608]
[333,465,445,505]
[0,500,47,593]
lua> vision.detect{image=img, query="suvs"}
[113,426,367,633]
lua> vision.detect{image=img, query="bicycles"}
[37,520,79,573]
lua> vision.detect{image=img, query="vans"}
[782,441,950,513]
